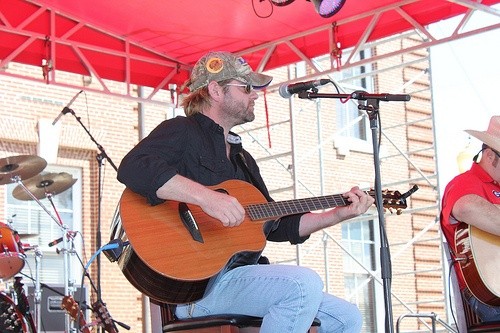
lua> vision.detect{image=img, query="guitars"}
[90,298,120,333]
[61,294,92,333]
[105,176,409,307]
[454,219,500,308]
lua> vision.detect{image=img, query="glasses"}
[218,82,253,94]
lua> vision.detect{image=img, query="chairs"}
[460,290,500,333]
[157,301,320,333]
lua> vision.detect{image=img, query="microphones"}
[279,79,331,99]
[52,90,84,125]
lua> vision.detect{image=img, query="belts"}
[464,289,473,300]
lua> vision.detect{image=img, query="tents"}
[0,1,499,333]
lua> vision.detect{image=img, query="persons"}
[116,50,378,331]
[438,115,500,333]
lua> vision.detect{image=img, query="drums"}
[0,221,28,282]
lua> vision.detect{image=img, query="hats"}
[189,51,273,90]
[464,116,500,152]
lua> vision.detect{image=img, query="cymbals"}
[0,154,49,186]
[11,170,80,202]
